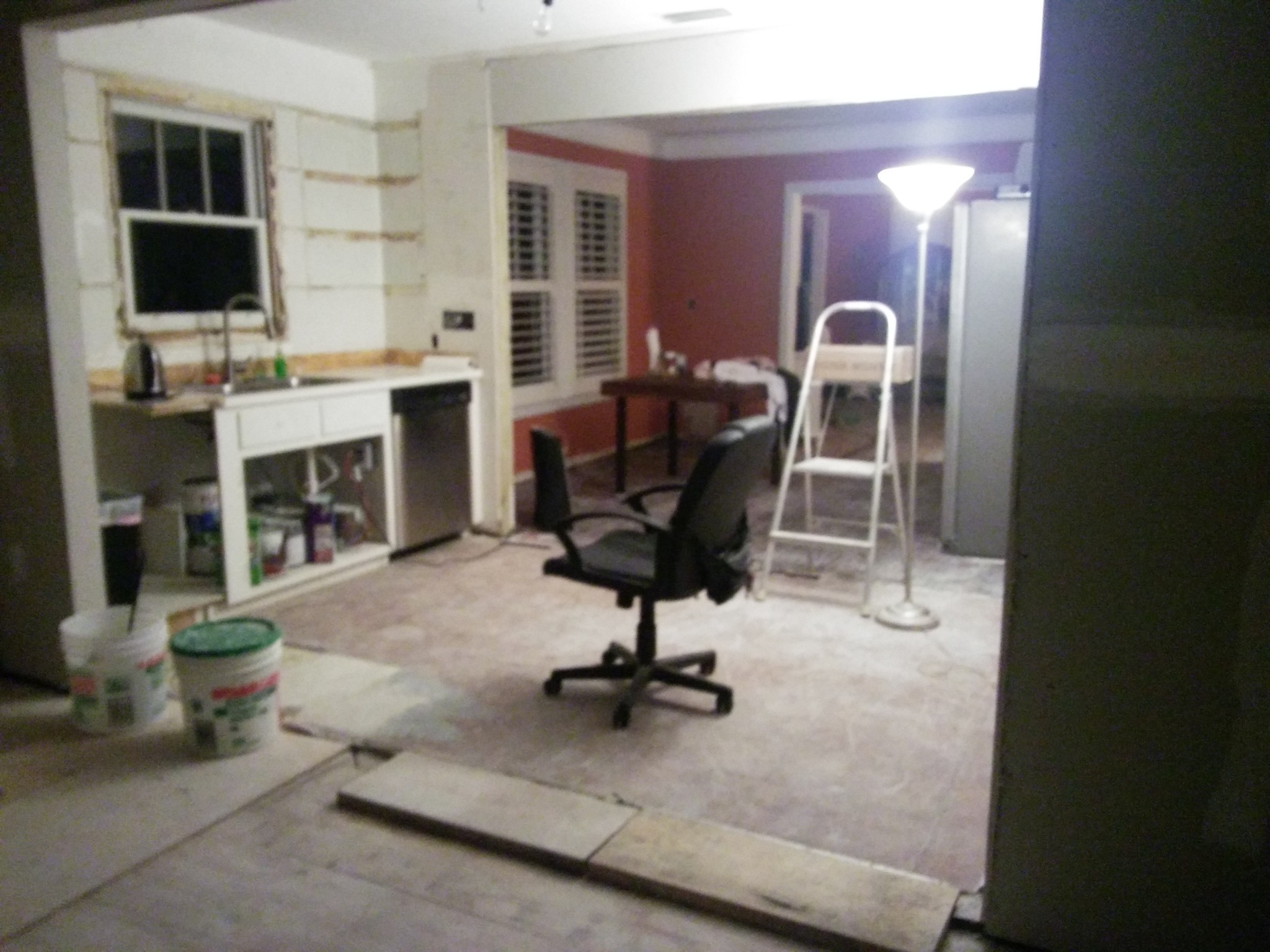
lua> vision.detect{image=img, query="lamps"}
[868,156,976,630]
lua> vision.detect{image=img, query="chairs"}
[543,409,778,729]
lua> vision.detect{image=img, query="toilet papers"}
[644,327,661,372]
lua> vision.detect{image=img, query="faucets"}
[223,294,274,388]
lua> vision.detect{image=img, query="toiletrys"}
[271,345,288,379]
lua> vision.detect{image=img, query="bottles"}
[275,346,288,377]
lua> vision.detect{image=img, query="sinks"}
[186,376,356,397]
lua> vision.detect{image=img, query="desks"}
[598,368,804,501]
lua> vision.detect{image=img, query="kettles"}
[124,333,166,401]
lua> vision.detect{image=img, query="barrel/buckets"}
[170,616,281,757]
[58,604,167,735]
